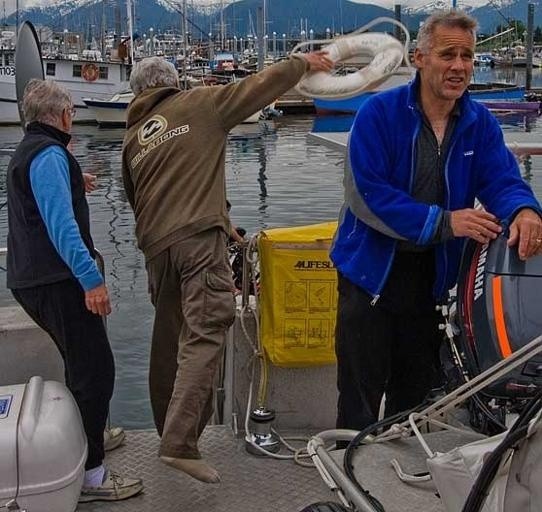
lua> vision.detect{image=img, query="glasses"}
[68,107,76,118]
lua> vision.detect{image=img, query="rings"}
[536,239,541,243]
[320,59,324,62]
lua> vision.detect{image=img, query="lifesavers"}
[82,63,98,81]
[294,32,405,100]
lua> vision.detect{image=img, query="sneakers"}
[79,469,144,501]
[104,426,124,451]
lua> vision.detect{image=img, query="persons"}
[121,50,336,484]
[5,78,142,502]
[329,11,542,451]
